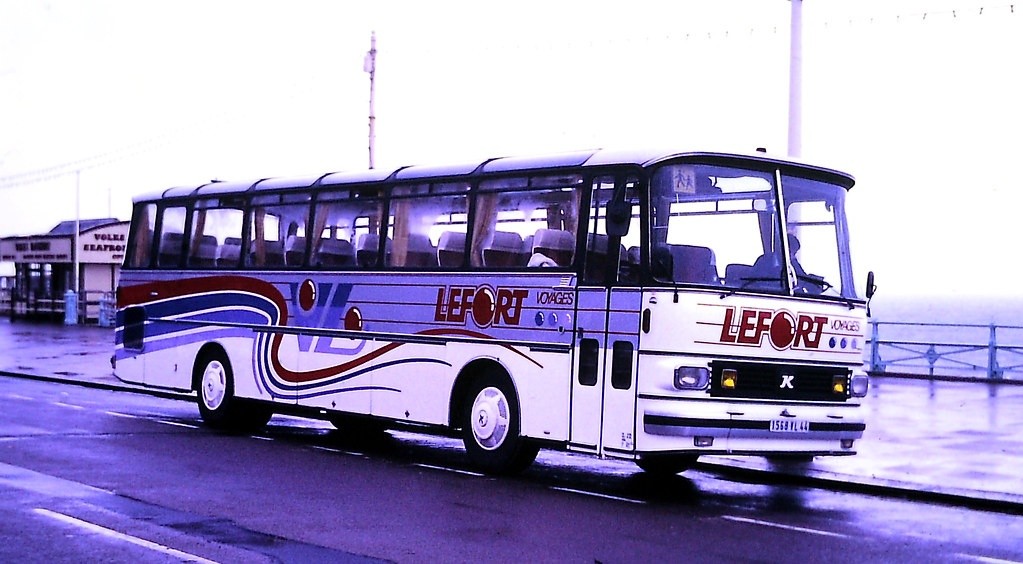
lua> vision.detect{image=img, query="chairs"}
[152,228,754,290]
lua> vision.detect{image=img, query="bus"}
[110,148,878,476]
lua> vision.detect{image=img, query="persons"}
[751,233,822,296]
